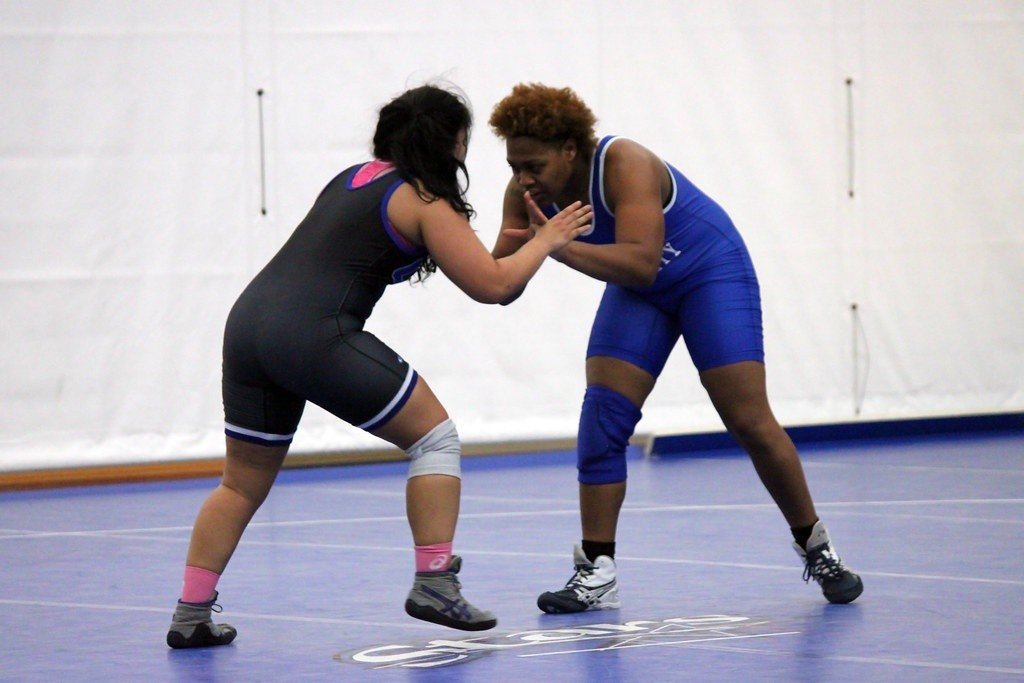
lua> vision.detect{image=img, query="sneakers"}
[405,555,497,631]
[792,518,864,603]
[537,543,622,614]
[166,590,237,649]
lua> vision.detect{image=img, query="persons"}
[489,83,864,616]
[167,87,595,648]
